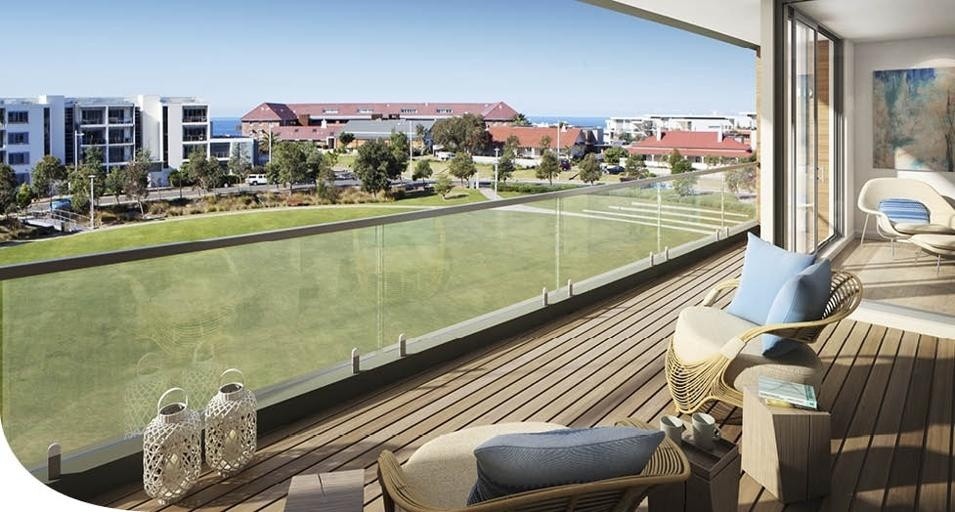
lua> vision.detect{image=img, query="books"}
[758,375,818,409]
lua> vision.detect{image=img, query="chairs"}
[664,266,863,415]
[375,419,692,512]
[857,176,955,277]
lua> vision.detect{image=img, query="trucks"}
[51,198,75,212]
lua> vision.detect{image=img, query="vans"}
[435,151,456,163]
[559,159,571,172]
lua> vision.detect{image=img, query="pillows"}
[724,232,818,327]
[466,424,666,505]
[762,255,832,358]
[877,199,930,224]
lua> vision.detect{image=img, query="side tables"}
[648,420,740,512]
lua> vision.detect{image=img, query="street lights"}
[86,174,97,231]
[268,121,274,171]
[494,147,499,197]
[74,130,84,172]
[555,120,568,182]
[396,120,414,181]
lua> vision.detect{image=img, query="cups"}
[691,409,724,450]
[660,415,685,447]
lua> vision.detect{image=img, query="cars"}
[208,170,355,189]
[606,166,626,175]
[654,182,672,190]
